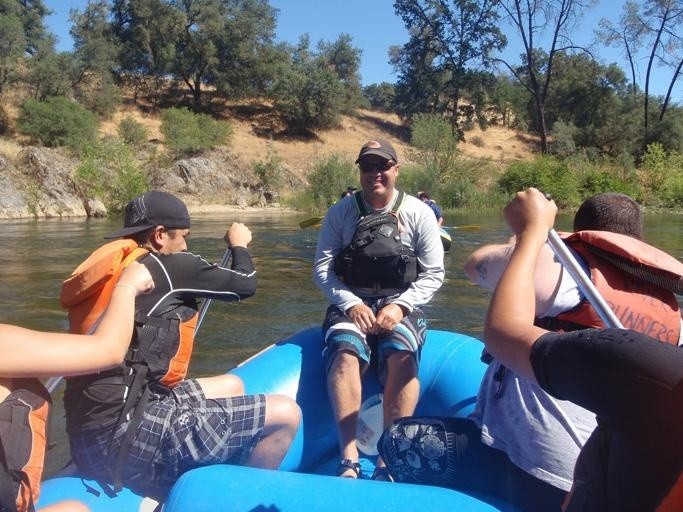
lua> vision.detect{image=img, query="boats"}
[439,228,452,252]
[29,326,516,512]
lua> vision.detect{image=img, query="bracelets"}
[113,282,139,298]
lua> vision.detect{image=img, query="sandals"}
[335,457,394,482]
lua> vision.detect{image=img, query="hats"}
[102,190,192,239]
[355,137,398,167]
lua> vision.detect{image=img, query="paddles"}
[441,226,481,232]
[299,216,325,230]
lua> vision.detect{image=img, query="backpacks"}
[332,188,418,289]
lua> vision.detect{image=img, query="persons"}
[481,184,683,512]
[31,499,92,512]
[60,188,304,484]
[341,184,444,226]
[0,260,155,511]
[375,190,683,512]
[312,138,446,479]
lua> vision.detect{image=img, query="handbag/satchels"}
[375,410,482,485]
